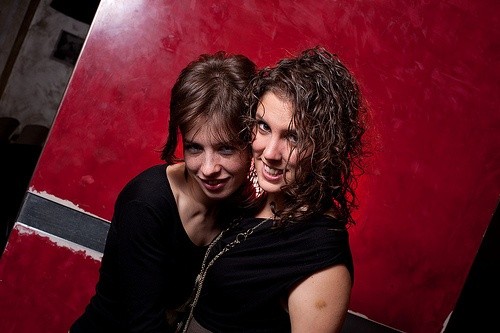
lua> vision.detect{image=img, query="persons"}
[174,46,365,333]
[66,51,263,333]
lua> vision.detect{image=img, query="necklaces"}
[181,200,275,333]
